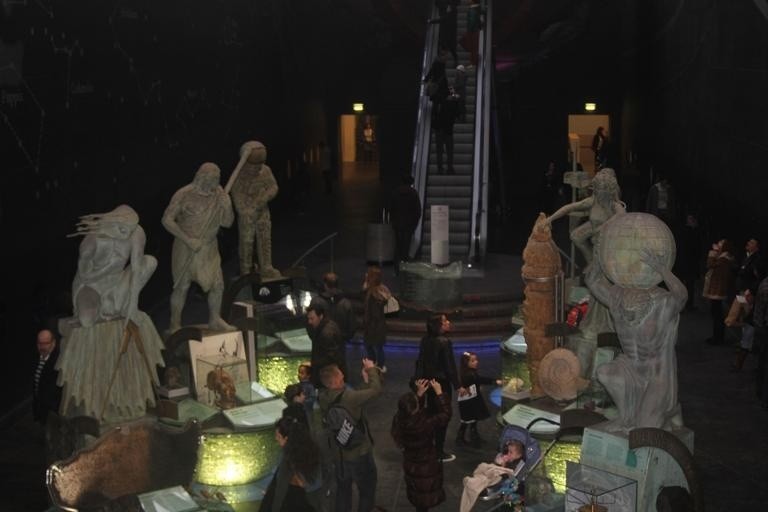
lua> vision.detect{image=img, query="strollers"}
[457,416,563,512]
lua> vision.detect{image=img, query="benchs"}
[39,416,199,511]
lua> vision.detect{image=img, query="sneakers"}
[707,334,721,348]
[438,452,457,464]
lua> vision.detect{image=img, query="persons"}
[318,141,333,193]
[544,165,626,266]
[231,141,280,278]
[424,0,482,175]
[68,205,160,327]
[388,177,422,277]
[30,330,62,423]
[161,162,238,332]
[585,249,688,431]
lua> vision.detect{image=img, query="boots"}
[735,348,748,370]
[455,431,487,446]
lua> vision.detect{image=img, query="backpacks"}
[322,387,369,454]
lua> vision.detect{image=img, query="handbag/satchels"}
[414,350,426,379]
